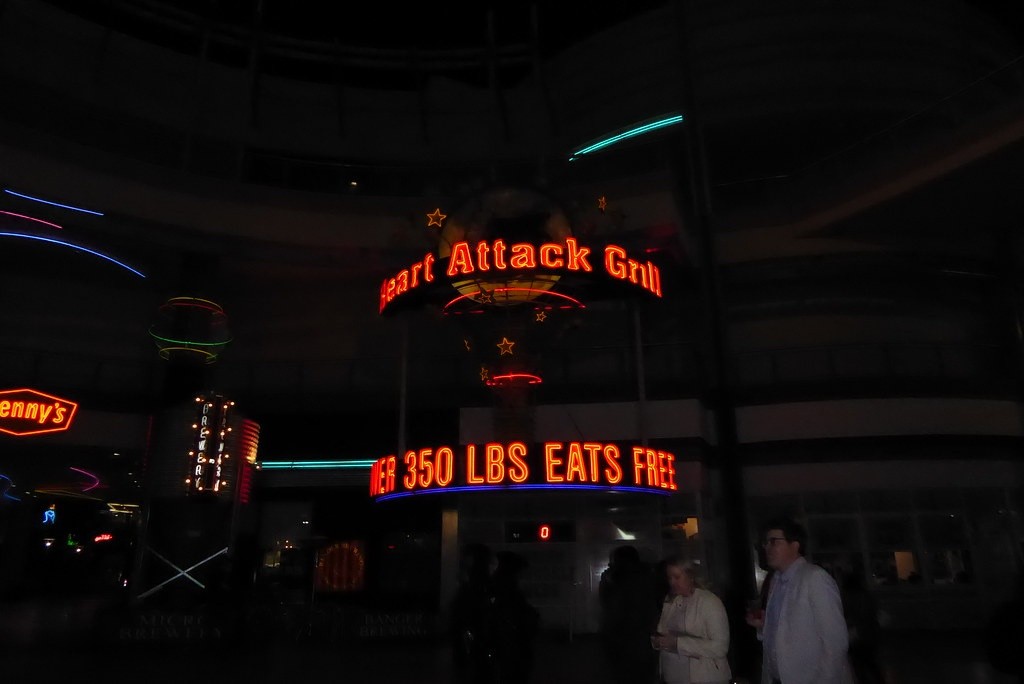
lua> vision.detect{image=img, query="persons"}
[599,546,671,684]
[745,520,853,684]
[448,542,541,684]
[844,560,886,684]
[650,552,732,684]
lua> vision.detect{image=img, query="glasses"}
[765,537,786,546]
